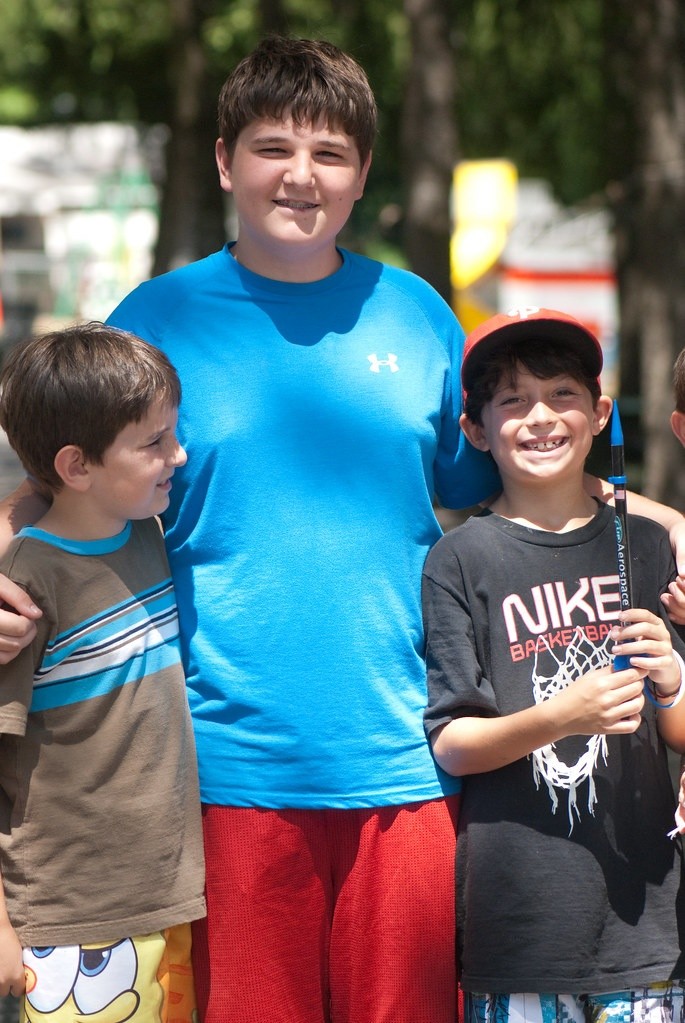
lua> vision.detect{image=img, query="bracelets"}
[641,650,685,708]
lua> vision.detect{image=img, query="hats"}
[460,308,603,410]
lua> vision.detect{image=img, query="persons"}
[422,308,685,1023]
[0,321,209,1023]
[0,37,685,1023]
